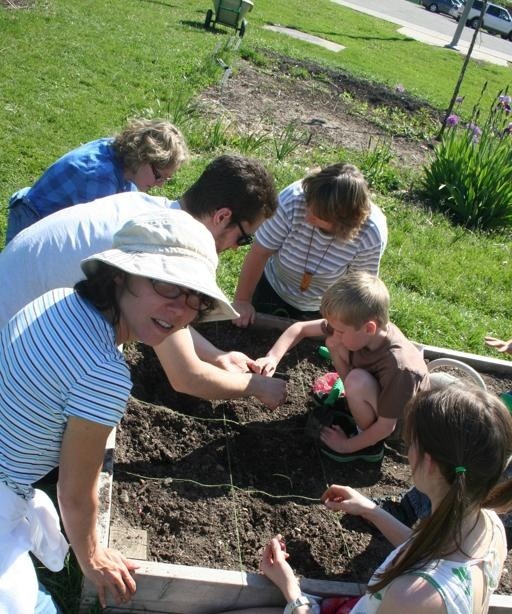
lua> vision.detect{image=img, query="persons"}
[259,380,512,614]
[0,209,240,614]
[228,162,388,329]
[6,118,189,246]
[253,271,431,464]
[0,155,288,411]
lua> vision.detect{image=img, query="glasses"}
[237,217,253,248]
[148,276,212,314]
[148,162,170,186]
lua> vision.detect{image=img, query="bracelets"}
[288,596,310,614]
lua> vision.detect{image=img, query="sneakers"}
[320,429,385,463]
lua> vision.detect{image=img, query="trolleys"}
[204,0,254,38]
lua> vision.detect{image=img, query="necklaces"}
[300,224,336,291]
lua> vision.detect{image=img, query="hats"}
[78,207,241,325]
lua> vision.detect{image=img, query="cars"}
[422,0,462,19]
[456,0,512,42]
